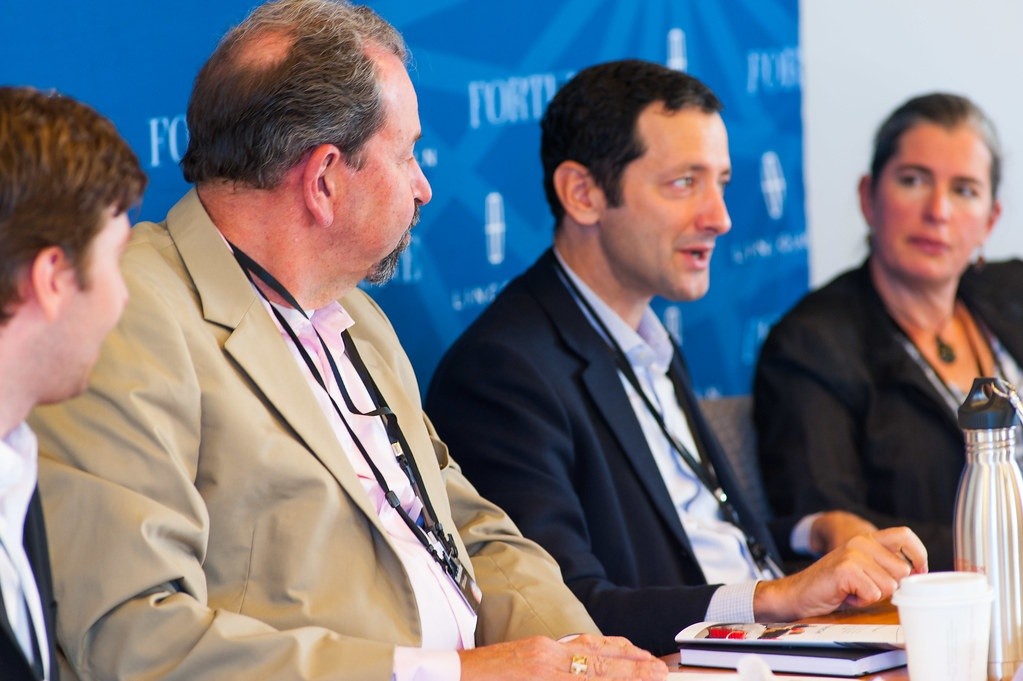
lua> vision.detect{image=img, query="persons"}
[754,94,1022,593]
[422,59,931,650]
[26,0,672,680]
[0,87,150,681]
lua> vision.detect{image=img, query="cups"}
[890,569,997,681]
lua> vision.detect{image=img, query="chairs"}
[696,396,793,586]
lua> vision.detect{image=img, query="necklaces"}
[885,296,962,368]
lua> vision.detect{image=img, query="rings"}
[572,653,588,674]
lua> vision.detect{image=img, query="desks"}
[655,602,912,681]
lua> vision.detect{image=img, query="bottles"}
[954,377,1022,681]
